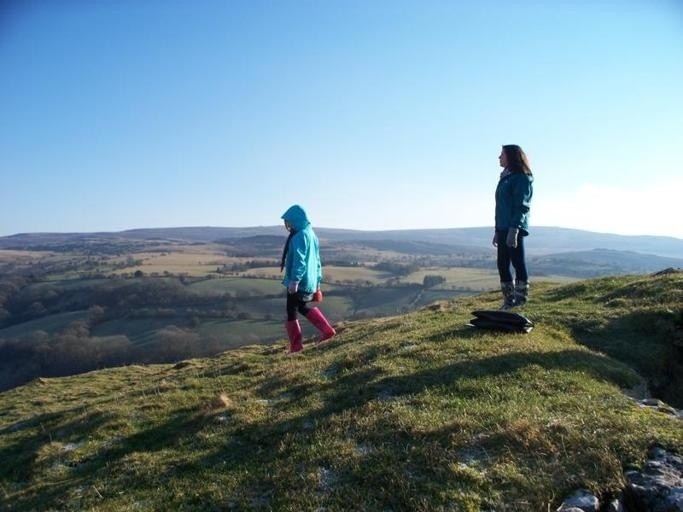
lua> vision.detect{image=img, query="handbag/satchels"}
[470,310,534,333]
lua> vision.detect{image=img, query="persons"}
[279,205,336,354]
[492,145,534,310]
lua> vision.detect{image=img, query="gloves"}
[506,228,518,248]
[287,281,298,293]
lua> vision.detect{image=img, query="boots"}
[513,280,528,307]
[499,281,515,311]
[285,320,303,353]
[304,307,336,344]
